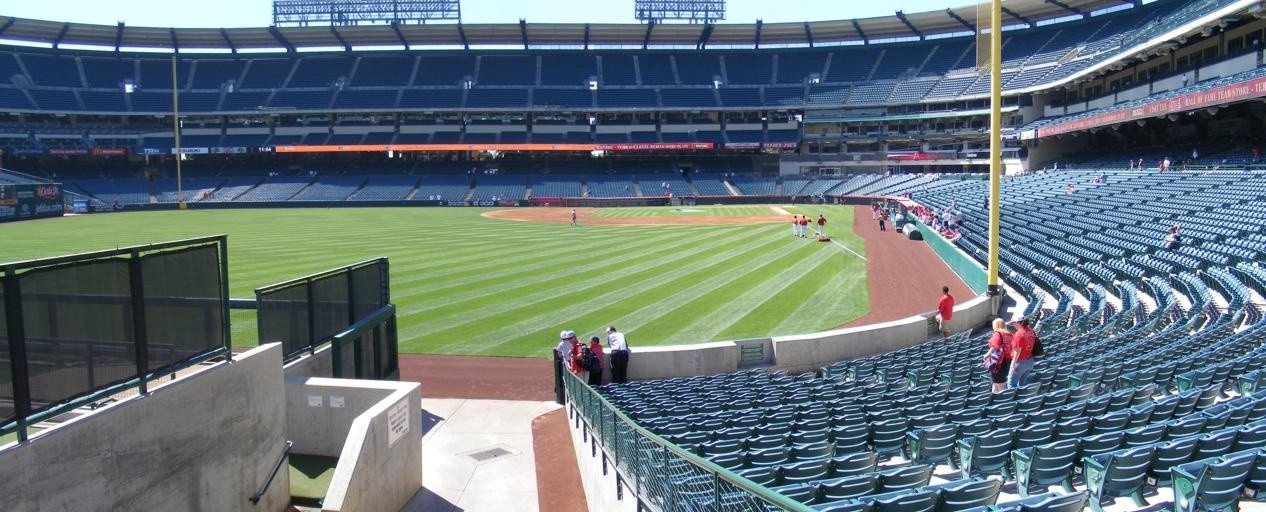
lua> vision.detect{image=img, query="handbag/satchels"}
[577,344,600,371]
[984,350,1004,373]
[1032,337,1044,356]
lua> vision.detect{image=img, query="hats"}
[561,330,576,339]
[606,326,615,333]
[1008,315,1024,324]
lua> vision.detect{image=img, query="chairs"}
[1001,265,1265,331]
[589,330,1266,509]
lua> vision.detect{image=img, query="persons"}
[1169,224,1180,241]
[871,191,963,244]
[557,331,603,386]
[791,191,843,238]
[606,326,629,384]
[1100,172,1107,183]
[1005,314,1035,389]
[983,196,988,209]
[1011,162,1057,181]
[1128,148,1199,171]
[984,318,1013,394]
[935,286,954,338]
